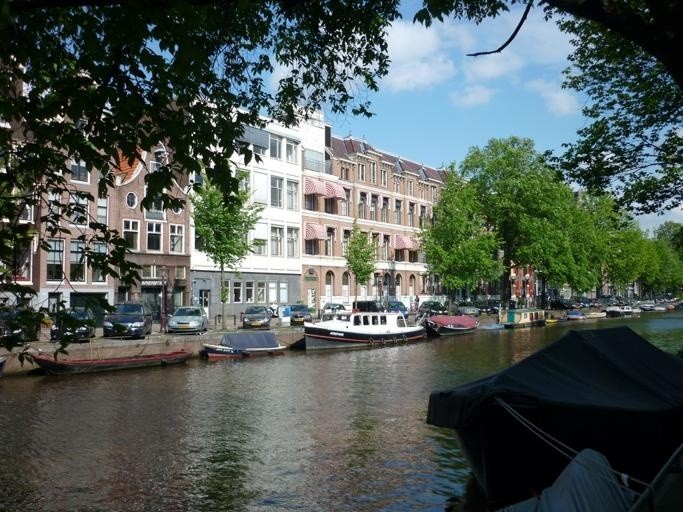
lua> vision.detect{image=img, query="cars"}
[550,296,601,310]
[0,309,37,341]
[320,300,408,319]
[164,306,208,333]
[50,307,96,342]
[102,302,153,338]
[241,304,312,329]
[419,300,522,317]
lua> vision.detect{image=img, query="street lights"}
[159,265,169,334]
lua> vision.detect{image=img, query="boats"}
[426,324,683,499]
[561,296,683,321]
[201,344,288,360]
[303,311,425,351]
[30,351,193,375]
[425,314,480,336]
[498,300,559,328]
